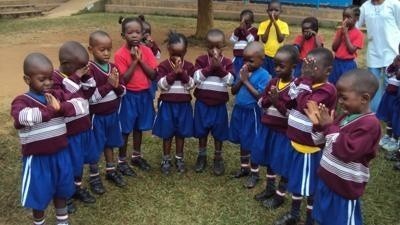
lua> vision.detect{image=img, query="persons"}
[11,0,400,225]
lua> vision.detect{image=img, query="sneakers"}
[54,155,314,225]
[379,135,400,172]
